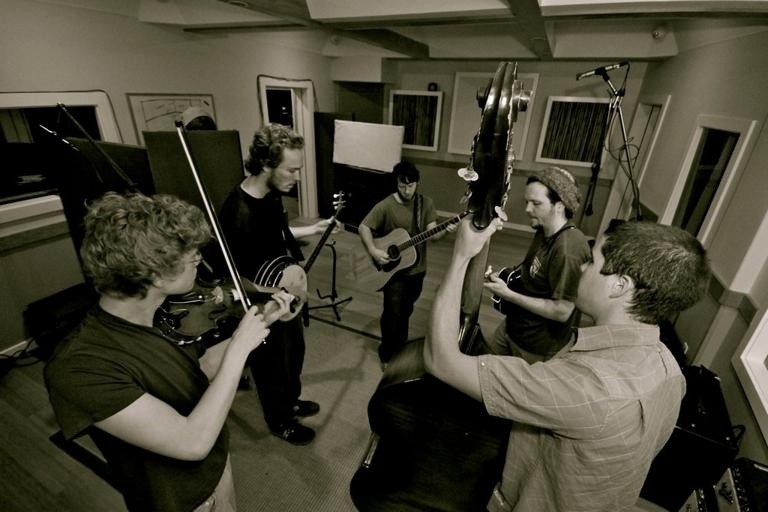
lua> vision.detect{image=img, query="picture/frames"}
[122,94,219,148]
[446,70,541,162]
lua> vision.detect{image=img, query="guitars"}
[353,211,470,291]
[485,263,522,315]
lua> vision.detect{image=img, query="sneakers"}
[270,421,316,448]
[298,398,321,417]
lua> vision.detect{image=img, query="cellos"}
[348,62,534,512]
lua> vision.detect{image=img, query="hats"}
[528,165,584,217]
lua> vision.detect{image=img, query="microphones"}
[576,62,620,80]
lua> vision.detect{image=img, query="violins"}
[154,275,300,346]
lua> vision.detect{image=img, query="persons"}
[42,192,294,512]
[421,206,713,512]
[481,165,592,364]
[212,122,345,445]
[357,161,460,371]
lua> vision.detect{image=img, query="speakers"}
[636,364,740,511]
